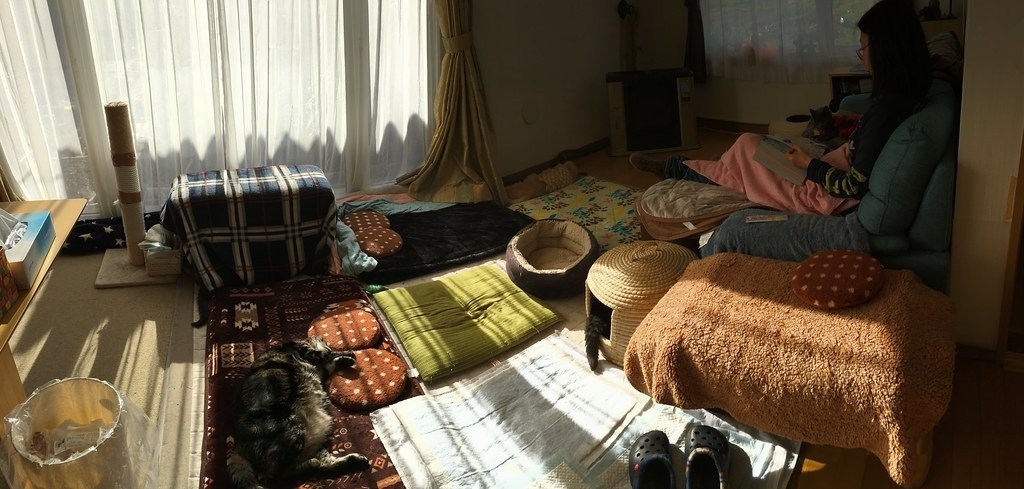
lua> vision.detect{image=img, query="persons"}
[630,0,932,217]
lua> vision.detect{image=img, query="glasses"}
[856,45,867,60]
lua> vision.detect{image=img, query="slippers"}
[686,424,728,489]
[629,430,674,489]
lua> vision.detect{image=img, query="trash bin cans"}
[10,377,134,489]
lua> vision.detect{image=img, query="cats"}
[585,299,612,371]
[232,332,368,489]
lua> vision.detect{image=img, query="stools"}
[623,252,956,489]
[159,165,338,292]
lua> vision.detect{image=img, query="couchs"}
[701,30,960,292]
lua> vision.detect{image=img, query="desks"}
[828,65,871,100]
[0,198,91,489]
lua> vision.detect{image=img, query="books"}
[753,128,826,185]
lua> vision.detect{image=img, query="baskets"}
[144,233,182,275]
